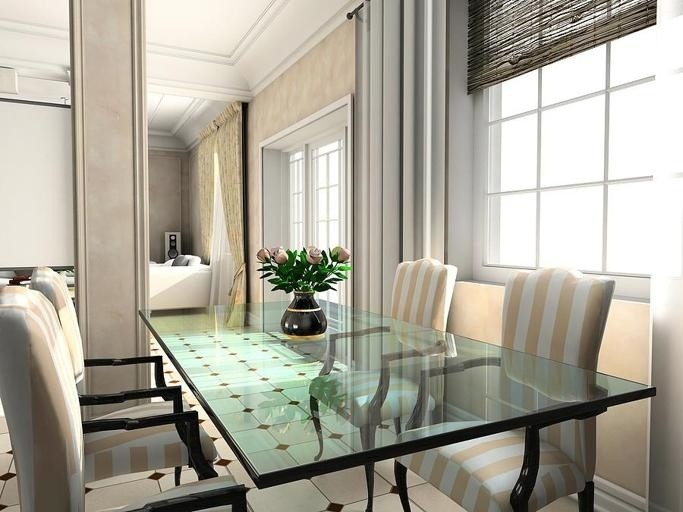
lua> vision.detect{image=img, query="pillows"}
[172,255,189,267]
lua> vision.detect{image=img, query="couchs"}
[147,255,211,317]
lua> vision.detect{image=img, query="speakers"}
[164,231,181,260]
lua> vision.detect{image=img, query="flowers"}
[256,244,351,293]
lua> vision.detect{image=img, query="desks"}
[137,297,655,512]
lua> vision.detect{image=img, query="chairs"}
[309,258,617,511]
[0,263,250,511]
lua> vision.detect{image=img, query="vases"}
[279,291,327,339]
